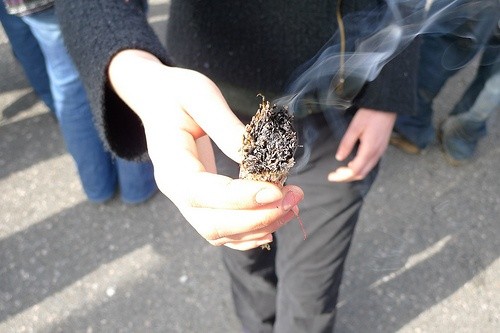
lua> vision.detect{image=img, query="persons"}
[0,0,159,206]
[390,1,500,167]
[55,0,428,333]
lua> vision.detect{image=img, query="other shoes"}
[440,114,464,165]
[388,128,424,155]
